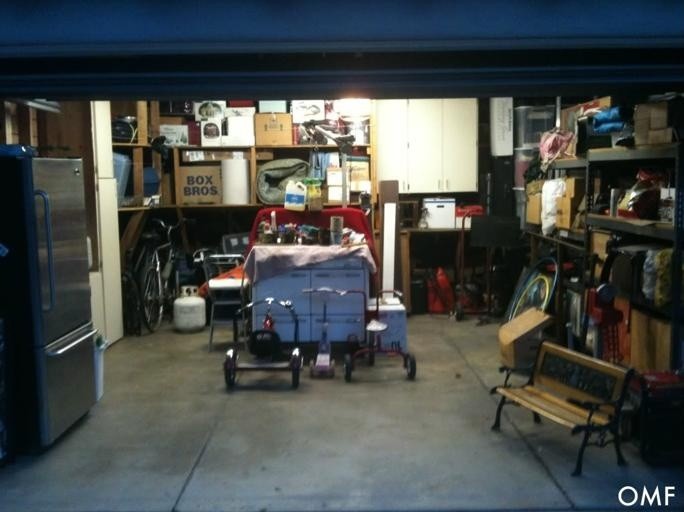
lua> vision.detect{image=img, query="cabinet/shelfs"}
[529,143,684,462]
[110,97,509,317]
[635,101,684,148]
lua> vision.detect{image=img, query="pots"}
[118,114,136,125]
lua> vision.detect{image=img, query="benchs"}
[489,339,634,477]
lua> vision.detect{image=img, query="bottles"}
[327,215,345,245]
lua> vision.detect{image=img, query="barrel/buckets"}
[284,179,308,211]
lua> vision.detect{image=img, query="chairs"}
[203,254,252,353]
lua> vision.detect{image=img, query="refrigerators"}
[0,154,97,451]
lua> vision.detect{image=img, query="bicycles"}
[141,217,187,333]
